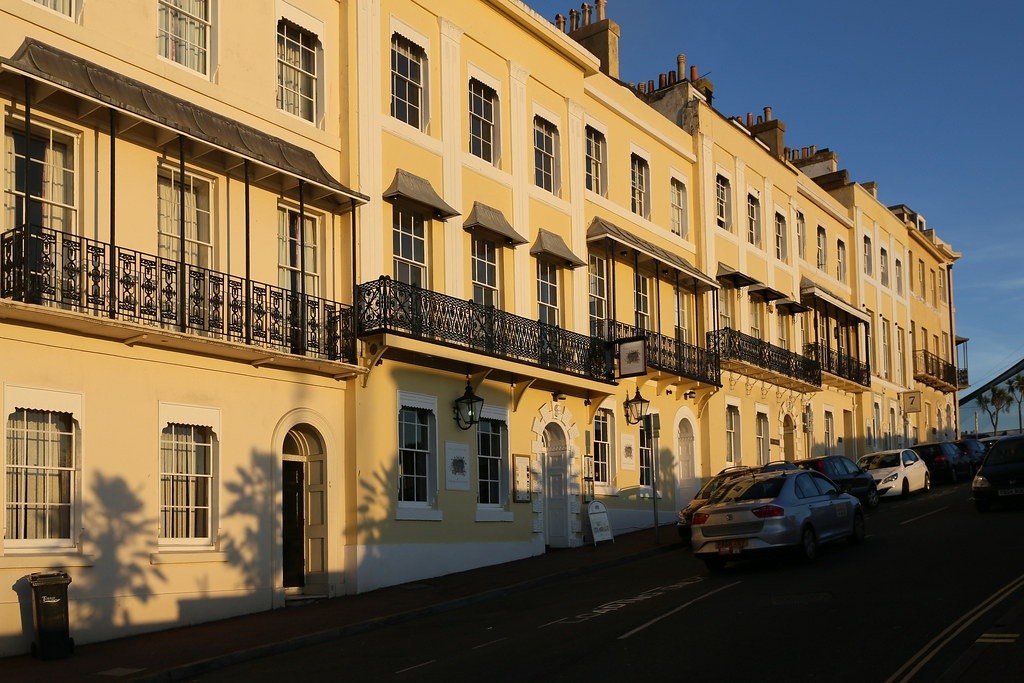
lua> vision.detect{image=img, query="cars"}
[791,455,879,508]
[856,449,932,501]
[977,436,1004,450]
[950,439,990,472]
[907,441,974,487]
[690,468,866,571]
[971,434,1024,509]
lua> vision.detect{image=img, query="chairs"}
[742,482,764,499]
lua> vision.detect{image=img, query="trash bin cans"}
[26,572,76,658]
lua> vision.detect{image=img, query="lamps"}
[453,380,484,430]
[624,385,650,425]
[834,327,837,339]
[684,391,696,399]
[552,390,566,400]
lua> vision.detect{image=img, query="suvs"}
[676,460,800,541]
